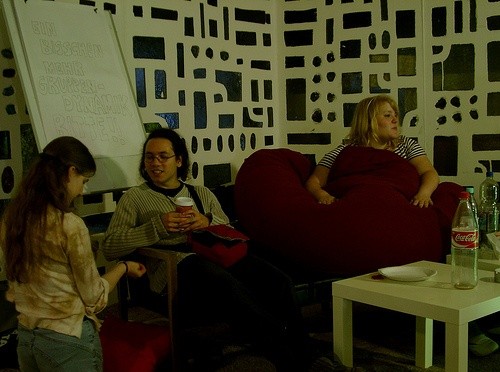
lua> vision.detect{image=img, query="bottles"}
[478,172,498,248]
[467,188,479,226]
[451,192,479,290]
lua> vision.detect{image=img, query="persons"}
[306,96,500,358]
[101,128,367,372]
[0,136,146,372]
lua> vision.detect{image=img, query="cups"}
[175,196,195,218]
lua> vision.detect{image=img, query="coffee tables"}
[332,254,500,372]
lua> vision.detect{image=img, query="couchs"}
[117,186,348,372]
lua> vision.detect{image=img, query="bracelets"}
[119,262,128,274]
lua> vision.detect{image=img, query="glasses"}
[142,153,177,163]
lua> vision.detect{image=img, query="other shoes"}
[468,333,500,357]
[487,326,500,334]
[309,356,370,372]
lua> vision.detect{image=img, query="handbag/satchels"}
[187,224,251,267]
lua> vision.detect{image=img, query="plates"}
[378,267,437,282]
